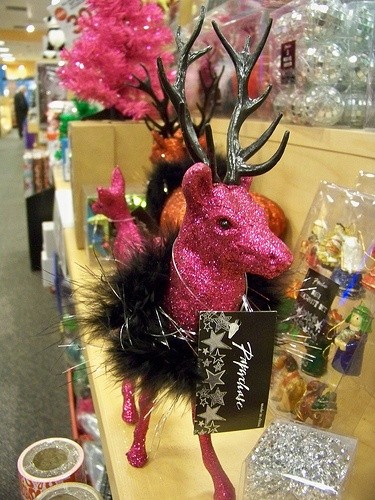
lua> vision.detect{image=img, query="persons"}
[13,88,27,139]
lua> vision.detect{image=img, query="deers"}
[37,5,292,500]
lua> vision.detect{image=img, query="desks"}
[53,164,375,500]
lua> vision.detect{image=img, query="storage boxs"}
[42,221,54,257]
[41,252,55,286]
[237,418,360,500]
